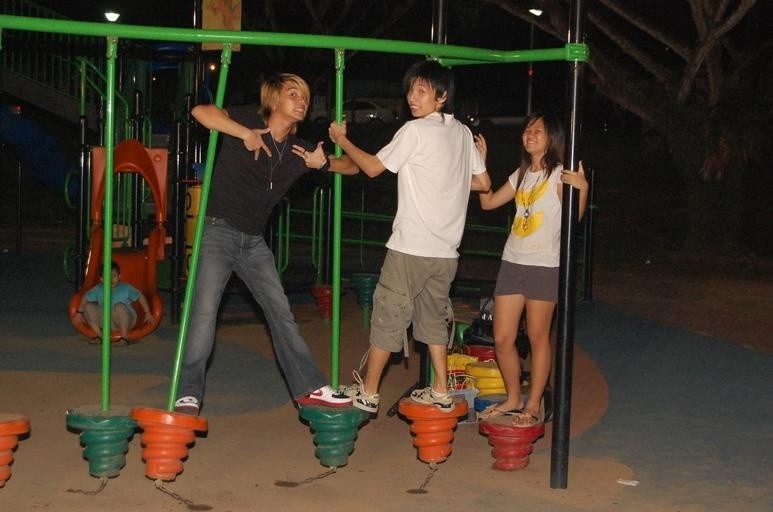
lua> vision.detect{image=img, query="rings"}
[302,148,307,155]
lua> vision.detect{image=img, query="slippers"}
[479,403,524,419]
[511,410,544,427]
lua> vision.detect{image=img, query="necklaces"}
[264,121,290,190]
[519,168,546,231]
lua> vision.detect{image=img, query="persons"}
[473,109,590,430]
[327,58,493,414]
[174,71,360,416]
[72,261,159,347]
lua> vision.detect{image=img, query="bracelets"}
[319,158,332,173]
[76,309,83,314]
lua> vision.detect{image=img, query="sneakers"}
[114,339,130,346]
[89,336,102,344]
[298,384,353,406]
[338,371,379,413]
[175,396,200,415]
[410,387,456,413]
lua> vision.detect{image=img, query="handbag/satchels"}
[463,313,529,359]
